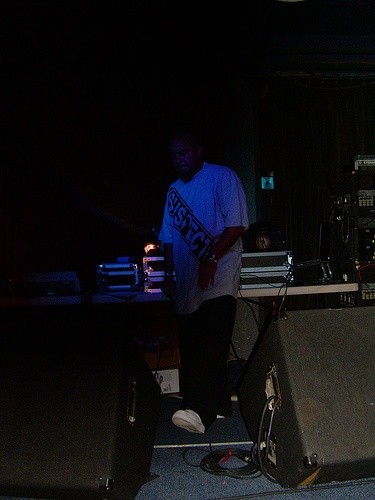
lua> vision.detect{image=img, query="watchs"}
[201,253,218,264]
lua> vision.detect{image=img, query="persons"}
[157,118,250,420]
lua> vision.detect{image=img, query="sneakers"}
[171,408,213,434]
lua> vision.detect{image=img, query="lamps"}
[254,232,272,250]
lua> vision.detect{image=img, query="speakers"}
[239,306,375,488]
[0,314,161,500]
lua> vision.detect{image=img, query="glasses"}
[170,146,196,156]
